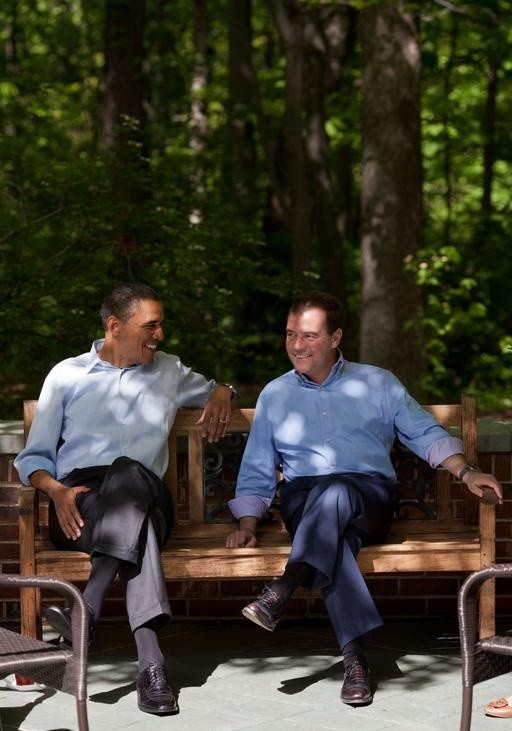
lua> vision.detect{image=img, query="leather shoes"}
[137,663,179,714]
[44,605,94,652]
[341,660,374,706]
[243,587,283,632]
[482,698,512,718]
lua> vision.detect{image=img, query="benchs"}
[13,394,500,651]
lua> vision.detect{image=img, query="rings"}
[220,419,226,424]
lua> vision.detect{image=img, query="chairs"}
[455,564,511,731]
[0,572,93,731]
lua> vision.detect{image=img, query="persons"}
[225,290,504,706]
[13,281,232,714]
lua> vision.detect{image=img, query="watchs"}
[223,383,237,400]
[459,464,481,482]
